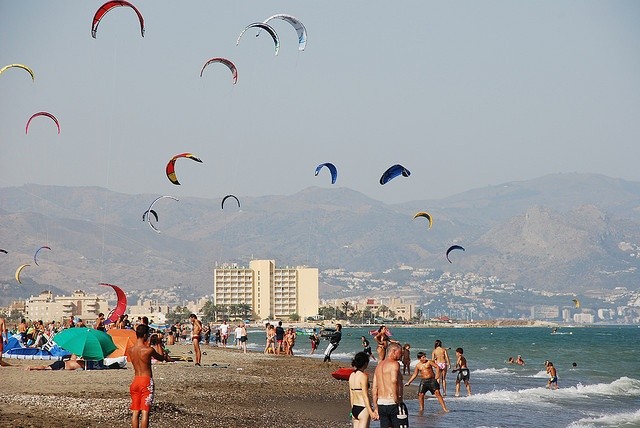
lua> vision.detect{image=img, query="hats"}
[223,320,226,325]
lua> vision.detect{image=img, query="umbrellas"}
[52,327,118,371]
[106,329,149,363]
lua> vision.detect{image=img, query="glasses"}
[406,346,410,350]
[381,328,386,331]
[423,356,426,358]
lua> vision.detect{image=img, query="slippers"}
[187,350,193,354]
[202,351,207,355]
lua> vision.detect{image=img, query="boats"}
[294,327,319,336]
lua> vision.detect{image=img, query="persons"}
[404,352,449,415]
[506,357,515,364]
[572,362,578,367]
[452,347,471,397]
[8,315,94,349]
[431,339,451,396]
[0,318,8,366]
[372,342,409,428]
[400,344,411,376]
[95,311,150,329]
[203,320,233,349]
[152,340,175,362]
[189,313,203,366]
[25,357,85,371]
[318,323,342,363]
[349,352,377,428]
[518,359,525,366]
[240,322,248,354]
[233,324,243,350]
[165,322,188,345]
[546,362,558,389]
[361,336,376,361]
[374,326,396,364]
[263,320,297,356]
[515,355,525,364]
[127,324,164,428]
[544,360,549,365]
[149,326,164,345]
[309,328,320,355]
